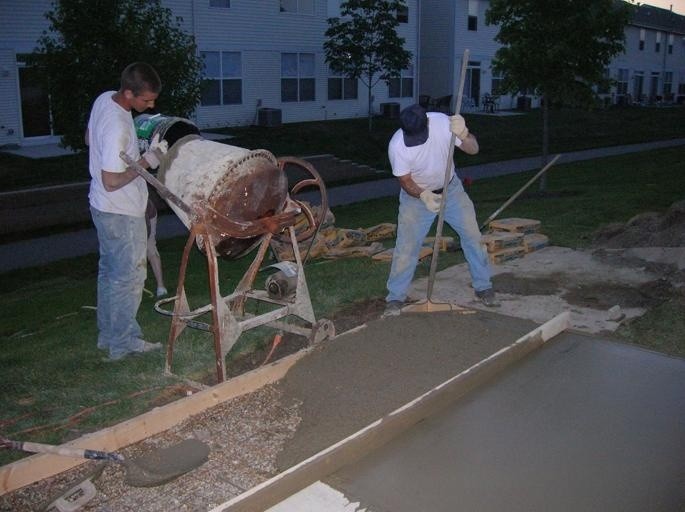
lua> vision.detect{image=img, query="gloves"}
[143,133,169,169]
[449,114,469,142]
[421,187,444,213]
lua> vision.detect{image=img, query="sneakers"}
[384,299,404,315]
[156,287,167,298]
[474,288,501,308]
[141,339,161,351]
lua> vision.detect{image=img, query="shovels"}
[0,433,210,487]
[445,154,561,252]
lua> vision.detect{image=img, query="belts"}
[432,175,454,192]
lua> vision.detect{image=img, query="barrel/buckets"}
[133,113,201,159]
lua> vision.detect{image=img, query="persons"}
[144,200,168,298]
[84,61,168,361]
[384,103,501,317]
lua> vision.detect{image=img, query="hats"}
[400,103,430,146]
